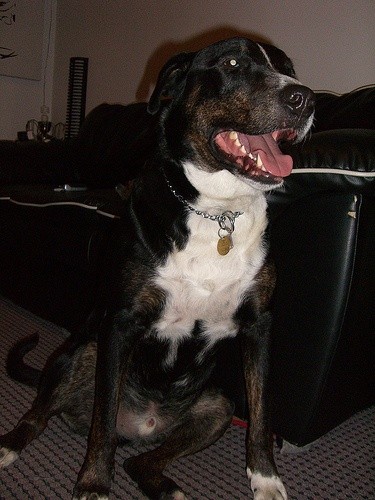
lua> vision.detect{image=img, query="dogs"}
[1,37,317,500]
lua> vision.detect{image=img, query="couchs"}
[1,83,375,450]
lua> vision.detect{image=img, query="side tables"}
[1,139,78,186]
